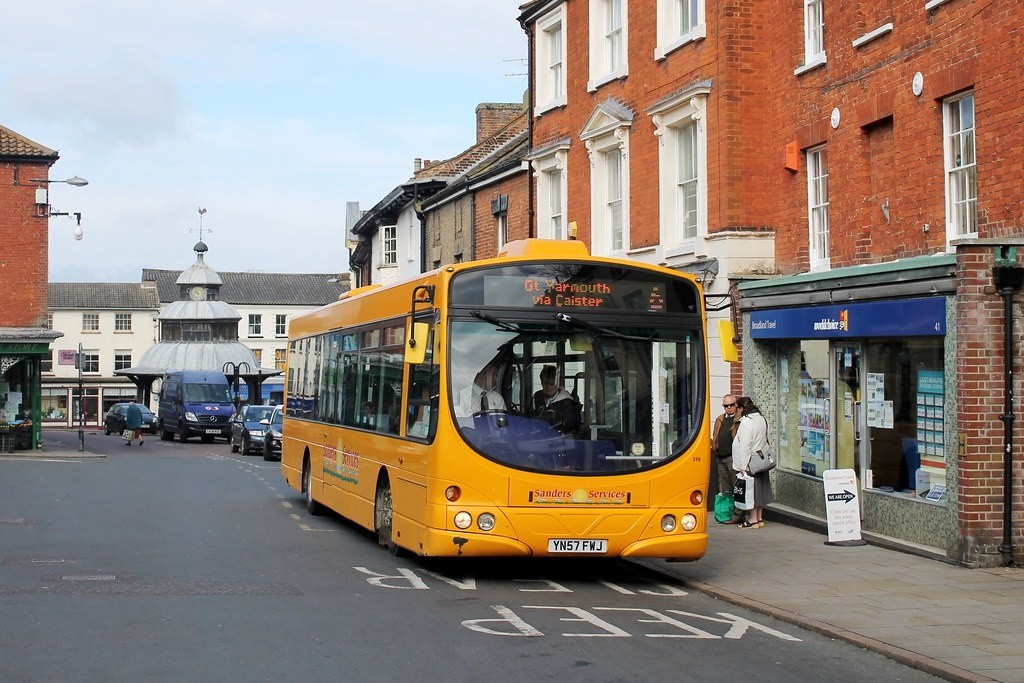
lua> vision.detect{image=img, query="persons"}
[126,401,144,446]
[533,365,583,435]
[712,394,741,524]
[459,365,507,418]
[731,397,774,529]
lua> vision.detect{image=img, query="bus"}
[282,238,741,563]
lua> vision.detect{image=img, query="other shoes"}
[126,443,131,445]
[138,440,143,446]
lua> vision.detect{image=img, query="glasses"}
[541,381,554,386]
[724,401,736,408]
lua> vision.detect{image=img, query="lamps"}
[31,212,83,241]
[29,176,89,187]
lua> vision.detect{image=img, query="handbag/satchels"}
[714,492,735,523]
[733,472,755,510]
[748,443,776,476]
[122,429,135,441]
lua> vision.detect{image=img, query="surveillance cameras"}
[75,227,84,240]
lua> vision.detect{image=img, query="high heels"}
[759,520,764,526]
[739,520,759,529]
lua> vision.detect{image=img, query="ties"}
[480,391,489,417]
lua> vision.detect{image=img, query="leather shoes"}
[724,518,736,524]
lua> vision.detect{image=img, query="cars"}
[104,403,159,436]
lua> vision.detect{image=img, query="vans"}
[158,370,236,444]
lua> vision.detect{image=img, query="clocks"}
[189,285,206,300]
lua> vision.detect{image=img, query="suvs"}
[229,403,273,455]
[260,404,283,460]
